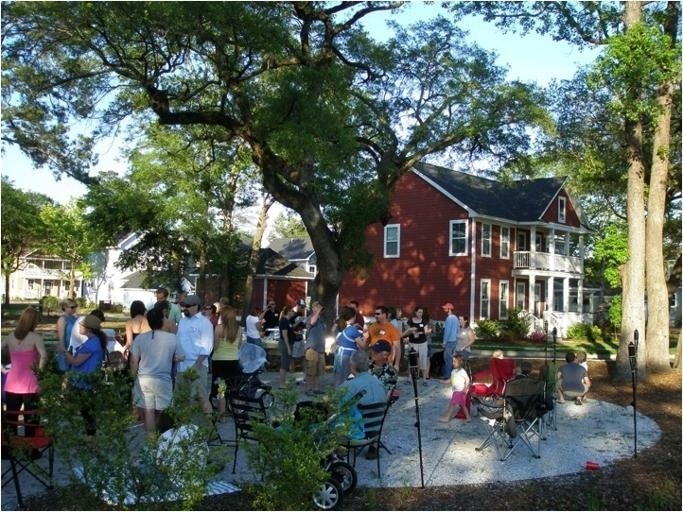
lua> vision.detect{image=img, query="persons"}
[349,300,364,331]
[129,308,185,441]
[207,303,217,327]
[121,300,151,379]
[303,300,327,397]
[556,352,591,405]
[245,306,264,374]
[515,361,532,380]
[287,298,307,383]
[154,301,177,335]
[333,307,369,386]
[217,296,229,325]
[480,395,504,413]
[368,305,402,372]
[67,309,106,371]
[174,295,215,423]
[415,334,431,379]
[437,354,473,423]
[438,303,460,384]
[576,351,588,372]
[455,313,474,362]
[263,301,279,337]
[208,305,241,411]
[277,304,295,390]
[56,298,78,390]
[63,315,107,435]
[403,307,429,387]
[395,308,409,361]
[386,307,398,329]
[364,339,400,461]
[330,349,387,440]
[1,307,47,462]
[156,287,183,326]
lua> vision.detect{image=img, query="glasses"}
[374,313,380,316]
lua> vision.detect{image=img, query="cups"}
[586,461,599,470]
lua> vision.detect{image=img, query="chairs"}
[227,388,283,485]
[347,382,401,481]
[1,407,56,492]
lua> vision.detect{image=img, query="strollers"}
[271,388,366,508]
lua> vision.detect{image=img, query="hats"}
[79,314,100,329]
[181,295,201,305]
[440,303,454,309]
[368,340,391,353]
